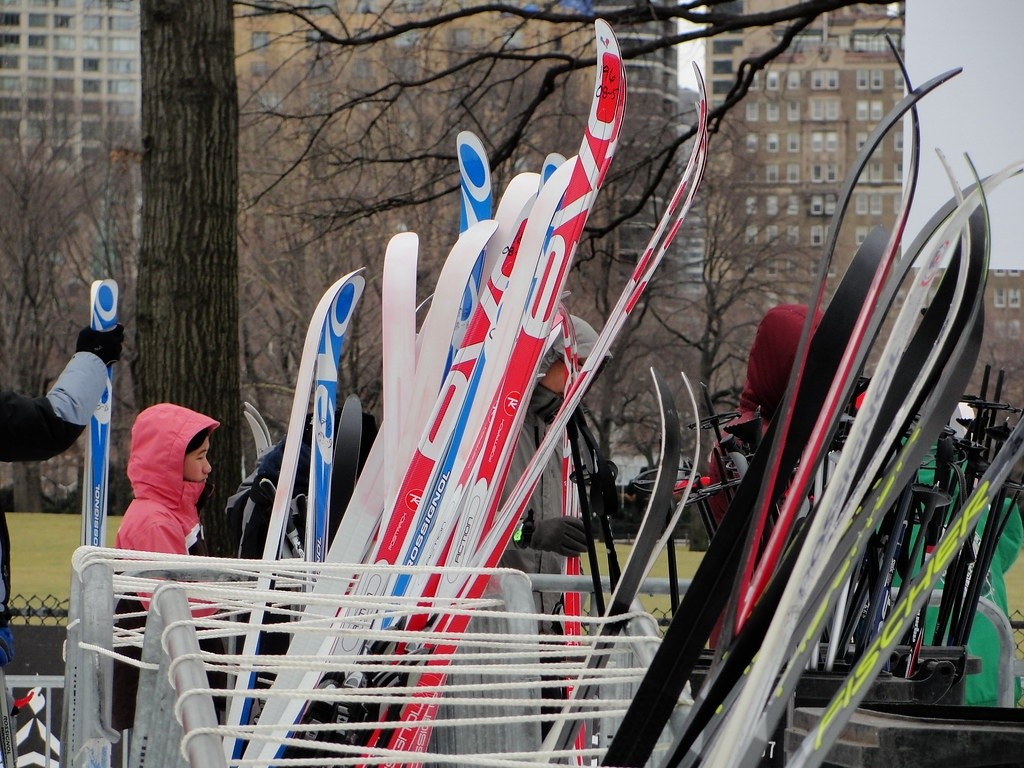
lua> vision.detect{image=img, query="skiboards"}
[43,18,1023,768]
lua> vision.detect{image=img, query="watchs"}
[498,311,612,744]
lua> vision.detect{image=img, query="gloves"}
[76,323,124,368]
[529,516,588,557]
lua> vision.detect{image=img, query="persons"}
[114,404,233,768]
[708,305,824,646]
[0,322,124,667]
